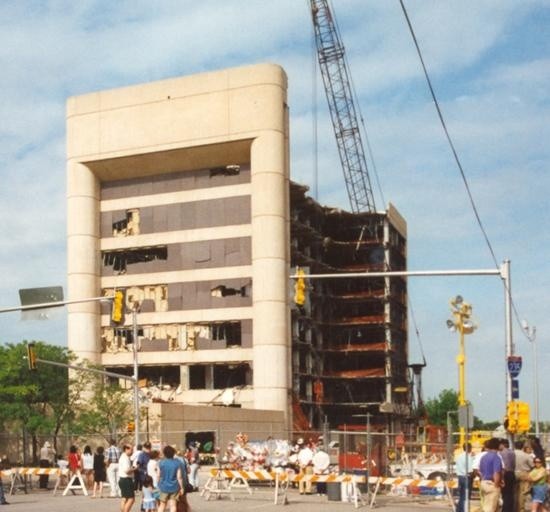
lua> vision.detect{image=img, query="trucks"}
[389,464,481,492]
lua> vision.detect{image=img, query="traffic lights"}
[128,423,134,431]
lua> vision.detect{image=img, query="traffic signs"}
[508,357,521,377]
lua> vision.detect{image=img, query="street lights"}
[446,290,478,453]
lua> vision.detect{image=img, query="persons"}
[56,439,122,498]
[454,437,549,512]
[118,441,201,512]
[216,440,330,495]
[39,441,56,488]
